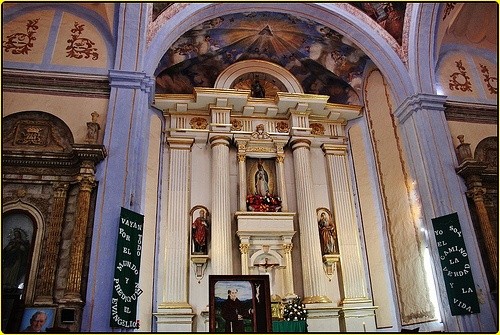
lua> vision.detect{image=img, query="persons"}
[318,211,337,254]
[24,311,49,334]
[84,110,103,144]
[192,209,209,254]
[254,160,270,196]
